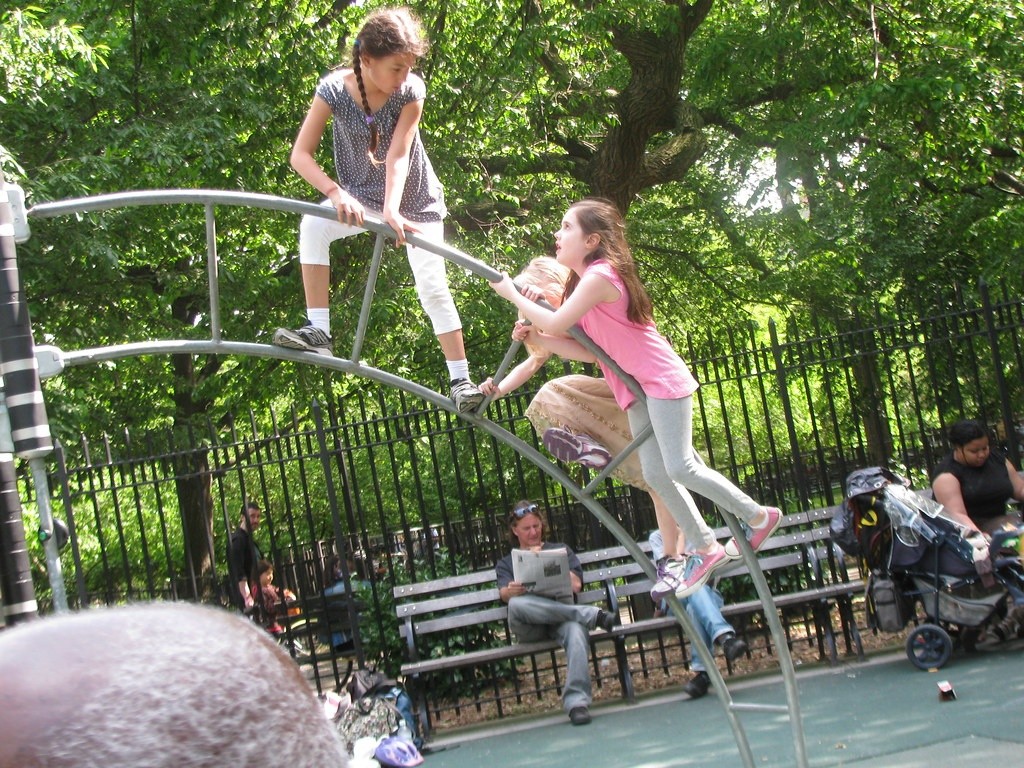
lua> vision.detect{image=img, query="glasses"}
[509,503,539,522]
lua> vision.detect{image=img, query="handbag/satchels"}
[346,668,400,714]
[872,577,911,633]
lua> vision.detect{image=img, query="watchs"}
[655,606,661,610]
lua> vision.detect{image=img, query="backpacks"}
[361,678,423,756]
[338,699,416,766]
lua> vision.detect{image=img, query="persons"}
[651,516,745,697]
[0,597,352,768]
[478,255,685,597]
[489,201,784,601]
[496,500,619,725]
[228,504,389,654]
[930,419,1024,642]
[274,11,485,412]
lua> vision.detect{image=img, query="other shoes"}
[723,638,751,674]
[682,671,710,698]
[569,707,591,725]
[596,610,617,632]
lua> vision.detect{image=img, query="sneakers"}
[674,542,730,599]
[649,552,694,603]
[724,506,784,560]
[450,377,486,414]
[271,325,334,358]
[543,424,613,470]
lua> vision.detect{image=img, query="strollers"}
[830,466,1024,671]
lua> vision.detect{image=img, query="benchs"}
[393,469,1024,739]
[241,589,368,700]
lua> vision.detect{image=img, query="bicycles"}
[245,604,353,695]
